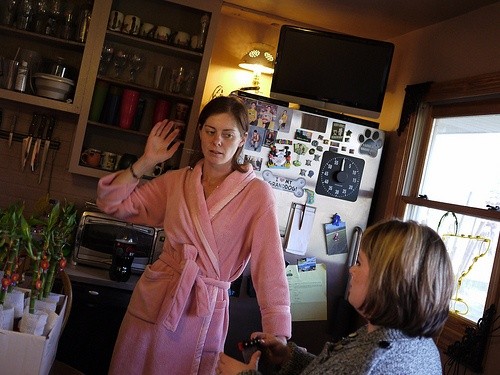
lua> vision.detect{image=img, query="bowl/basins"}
[34,72,74,100]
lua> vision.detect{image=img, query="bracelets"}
[127,164,144,181]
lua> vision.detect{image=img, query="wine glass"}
[97,45,144,85]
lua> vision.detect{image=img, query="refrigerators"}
[224,92,385,364]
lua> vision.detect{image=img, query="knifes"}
[8,111,56,184]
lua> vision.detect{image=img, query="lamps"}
[239,48,275,94]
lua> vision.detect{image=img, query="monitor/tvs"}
[269,24,395,119]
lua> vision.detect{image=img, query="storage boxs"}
[0,288,68,375]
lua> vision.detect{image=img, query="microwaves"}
[72,198,166,275]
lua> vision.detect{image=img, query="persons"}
[246,101,258,125]
[250,129,260,148]
[263,129,275,146]
[214,217,453,375]
[94,95,292,375]
[277,109,289,131]
[261,104,273,130]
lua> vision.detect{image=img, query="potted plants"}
[0,193,78,336]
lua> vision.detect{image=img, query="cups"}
[89,63,197,132]
[107,10,205,52]
[2,59,19,90]
[81,148,119,172]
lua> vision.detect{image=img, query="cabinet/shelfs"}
[0,0,224,189]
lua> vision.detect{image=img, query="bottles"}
[2,0,91,44]
[109,223,137,284]
[14,61,29,94]
[51,57,66,78]
